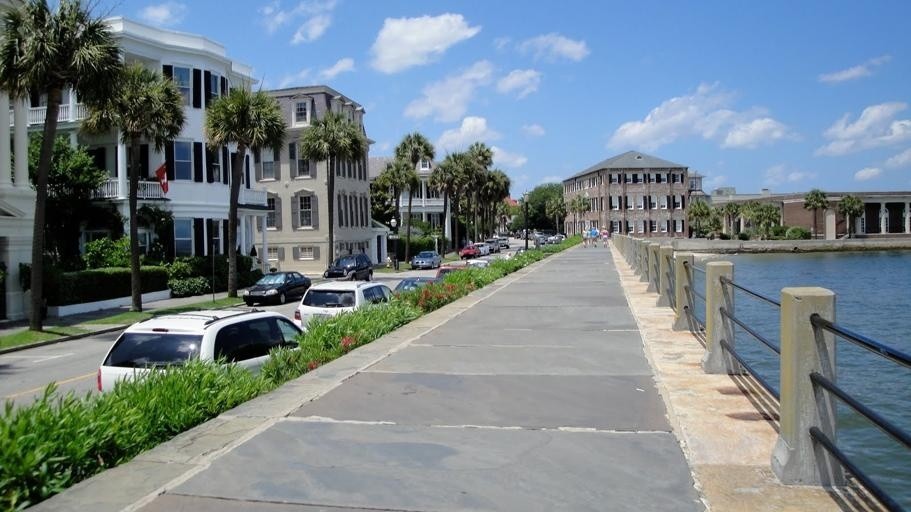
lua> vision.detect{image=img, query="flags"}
[155,163,170,194]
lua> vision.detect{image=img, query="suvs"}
[323,252,374,282]
[97,306,307,396]
[295,280,399,334]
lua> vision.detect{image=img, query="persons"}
[580,225,609,249]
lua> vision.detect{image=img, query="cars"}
[243,270,312,307]
[393,229,567,298]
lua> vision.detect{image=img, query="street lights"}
[390,215,401,270]
[521,188,530,249]
